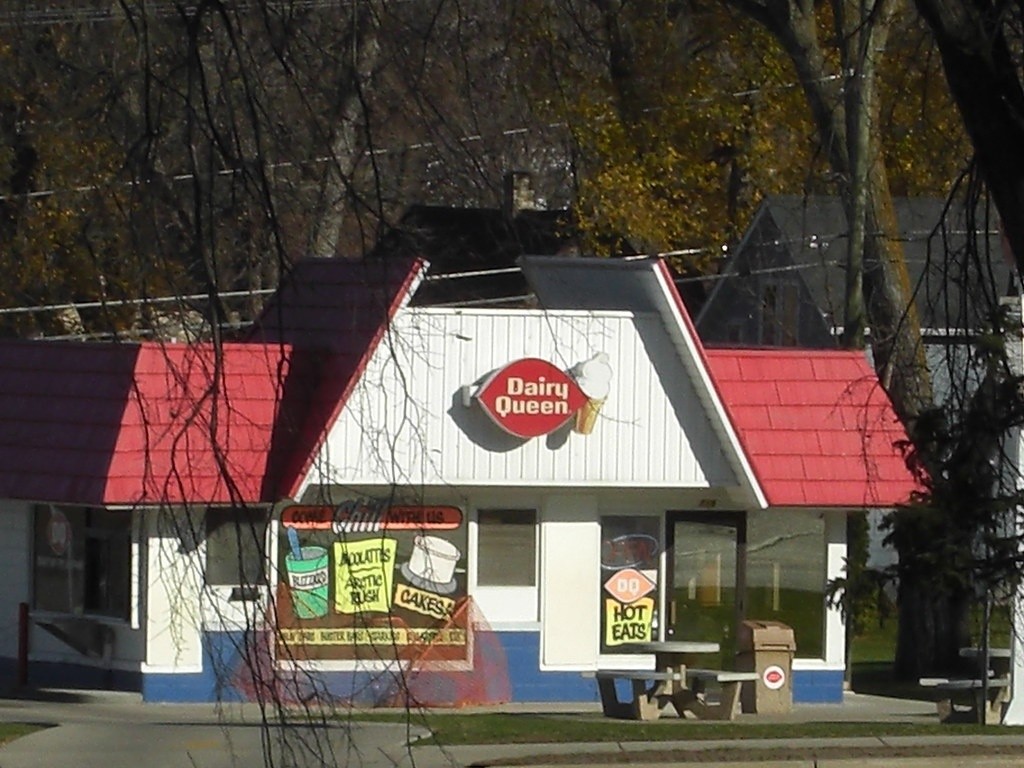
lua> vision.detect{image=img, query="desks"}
[625,641,720,719]
[959,648,1012,726]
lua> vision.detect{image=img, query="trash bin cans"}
[735,620,797,714]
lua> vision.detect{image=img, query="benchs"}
[685,669,761,720]
[596,669,680,719]
[921,678,1008,725]
